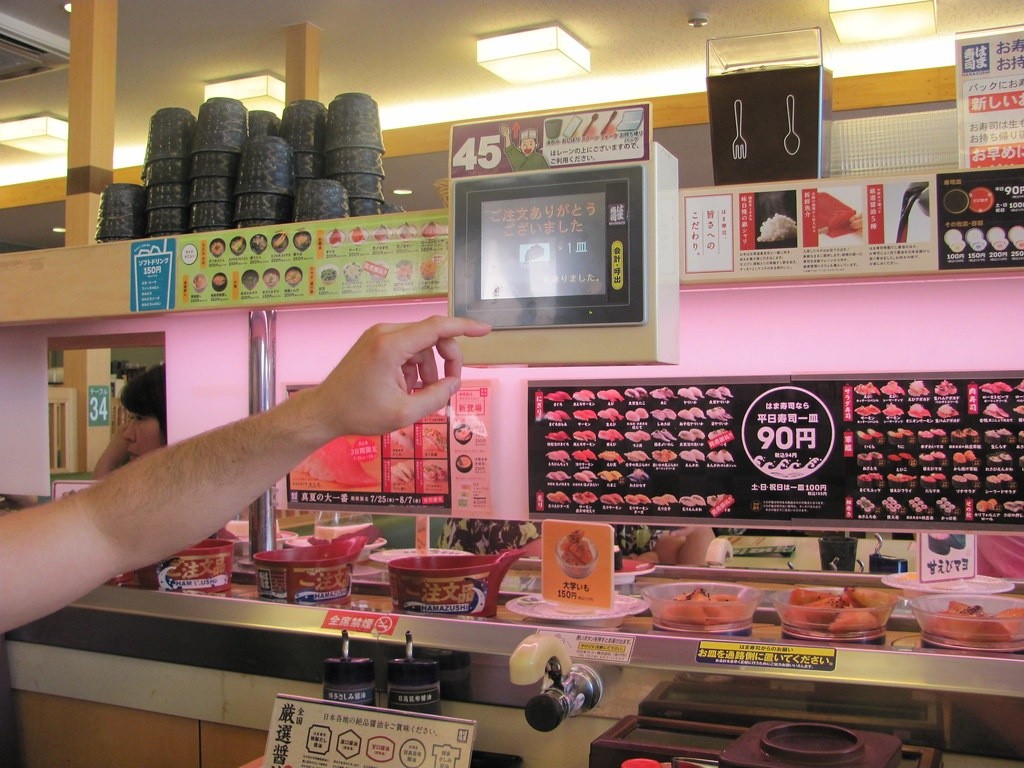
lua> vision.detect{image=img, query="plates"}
[369,548,475,564]
[276,530,387,561]
[505,593,649,620]
[614,558,656,585]
[881,571,1016,595]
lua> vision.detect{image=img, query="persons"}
[436,517,717,571]
[89,362,166,481]
[0,315,493,635]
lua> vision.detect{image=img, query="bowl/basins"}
[641,581,763,624]
[554,534,599,579]
[909,594,1024,644]
[139,538,234,598]
[772,585,900,634]
[253,535,370,609]
[387,549,527,620]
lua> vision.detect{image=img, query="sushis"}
[423,427,447,451]
[853,380,1024,518]
[391,430,414,449]
[422,463,448,481]
[391,462,413,482]
[295,437,380,486]
[543,386,734,508]
[928,533,966,556]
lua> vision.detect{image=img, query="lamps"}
[1,111,69,154]
[829,0,938,45]
[476,21,591,85]
[204,68,286,112]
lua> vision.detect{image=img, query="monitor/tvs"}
[453,163,649,329]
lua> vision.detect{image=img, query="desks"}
[715,535,918,573]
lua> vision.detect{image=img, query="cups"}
[95,91,409,245]
[818,536,858,572]
